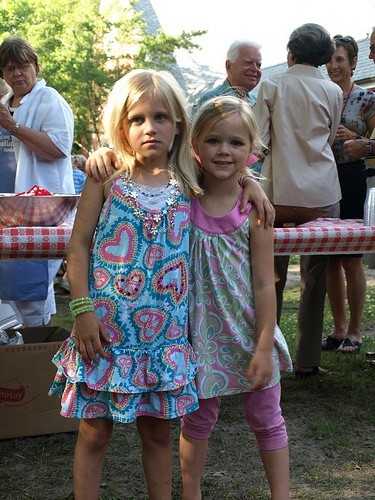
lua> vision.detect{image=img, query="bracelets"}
[69,297,95,319]
[240,175,258,188]
[356,133,360,139]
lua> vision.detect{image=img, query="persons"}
[343,25,375,162]
[317,32,375,354]
[0,36,91,331]
[83,96,296,500]
[249,21,344,378]
[47,69,278,499]
[185,39,268,181]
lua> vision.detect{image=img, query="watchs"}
[365,139,373,158]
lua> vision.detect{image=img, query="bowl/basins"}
[0,192,81,228]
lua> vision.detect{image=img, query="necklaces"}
[339,80,355,124]
[118,158,180,235]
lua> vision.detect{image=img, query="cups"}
[363,188,375,228]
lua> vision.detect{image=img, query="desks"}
[0,219,373,254]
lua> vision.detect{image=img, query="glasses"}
[368,43,375,51]
[0,64,33,73]
[332,36,358,55]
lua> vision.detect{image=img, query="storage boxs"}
[0,323,79,442]
